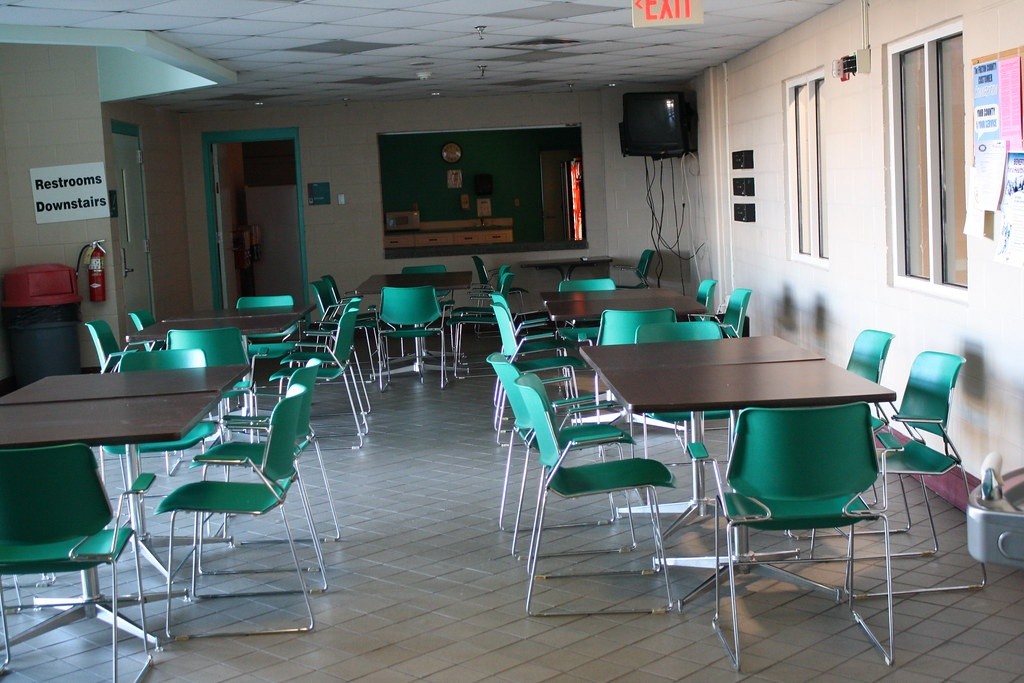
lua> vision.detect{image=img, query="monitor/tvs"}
[623,90,689,156]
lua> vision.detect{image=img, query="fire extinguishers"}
[75,239,107,301]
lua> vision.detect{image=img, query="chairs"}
[0,251,987,683]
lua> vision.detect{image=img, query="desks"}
[351,277,472,384]
[0,393,223,653]
[366,271,473,366]
[125,314,300,450]
[162,303,318,421]
[517,256,613,328]
[0,365,252,583]
[579,334,825,547]
[539,286,681,413]
[595,361,896,613]
[547,298,708,432]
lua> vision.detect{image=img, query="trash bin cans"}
[0,263,84,389]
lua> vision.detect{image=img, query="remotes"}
[580,257,588,261]
